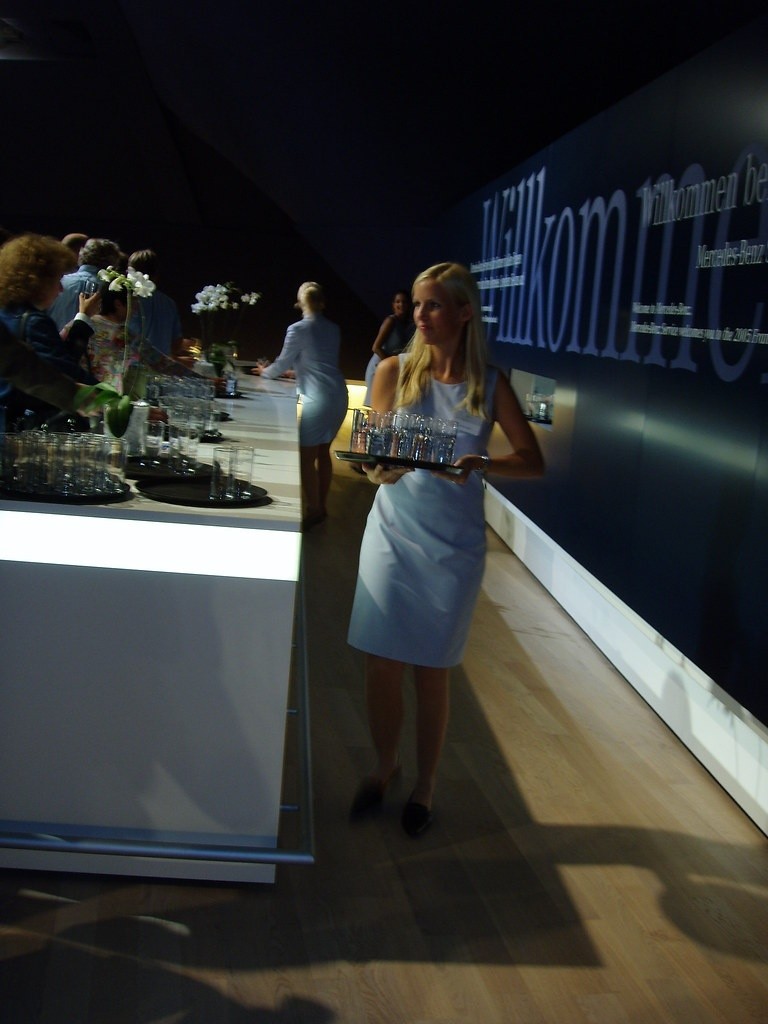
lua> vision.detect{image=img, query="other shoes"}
[350,781,384,820]
[402,788,433,838]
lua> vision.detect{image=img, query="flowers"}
[190,280,261,361]
[73,263,152,437]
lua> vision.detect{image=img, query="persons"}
[0,232,227,430]
[361,289,409,398]
[250,280,348,530]
[347,261,547,837]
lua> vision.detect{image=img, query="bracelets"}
[475,456,491,473]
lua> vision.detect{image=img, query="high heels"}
[302,511,326,531]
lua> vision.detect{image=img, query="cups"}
[144,375,253,500]
[350,408,459,462]
[525,393,550,420]
[84,280,99,298]
[0,429,128,495]
[258,357,269,367]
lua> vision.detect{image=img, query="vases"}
[100,398,150,457]
